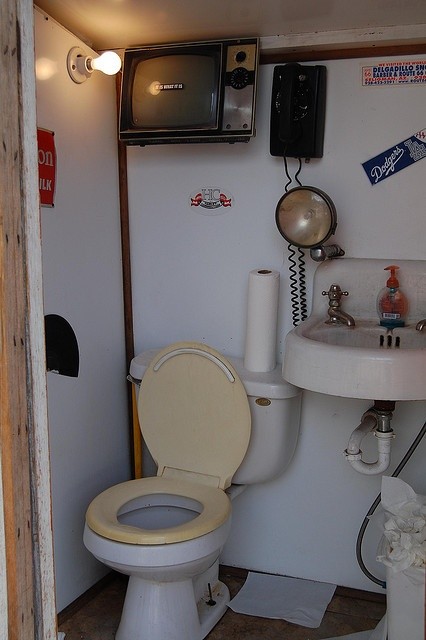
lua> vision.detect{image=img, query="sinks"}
[300,326,426,351]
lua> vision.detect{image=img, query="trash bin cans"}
[376,533,425,640]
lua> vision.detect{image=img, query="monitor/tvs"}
[118,34,261,146]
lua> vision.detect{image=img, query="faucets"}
[321,283,356,330]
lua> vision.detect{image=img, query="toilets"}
[82,340,304,640]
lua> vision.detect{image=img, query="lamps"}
[66,43,122,84]
